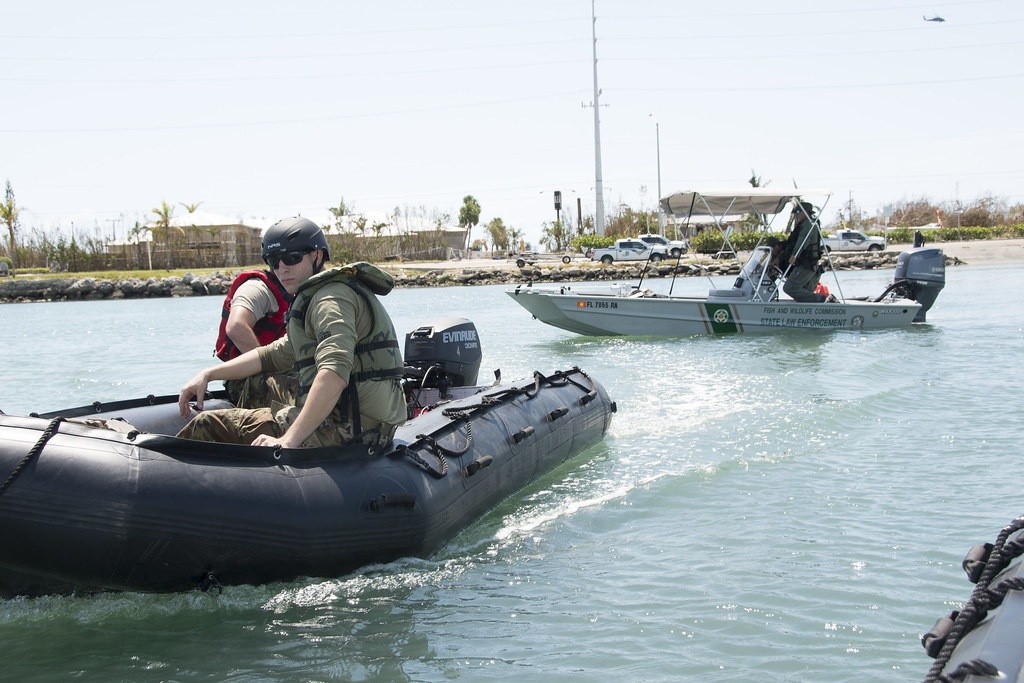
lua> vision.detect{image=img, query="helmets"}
[261,216,329,266]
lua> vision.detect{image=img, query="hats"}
[791,201,816,215]
[765,236,779,251]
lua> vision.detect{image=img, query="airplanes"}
[923,14,944,22]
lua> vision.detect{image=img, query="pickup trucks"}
[608,233,688,259]
[591,237,668,264]
[819,228,884,254]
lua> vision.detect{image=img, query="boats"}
[505,187,946,336]
[921,519,1024,683]
[0,319,617,597]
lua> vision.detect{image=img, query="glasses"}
[266,248,313,270]
[795,210,803,214]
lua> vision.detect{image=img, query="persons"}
[766,201,840,304]
[179,217,408,450]
